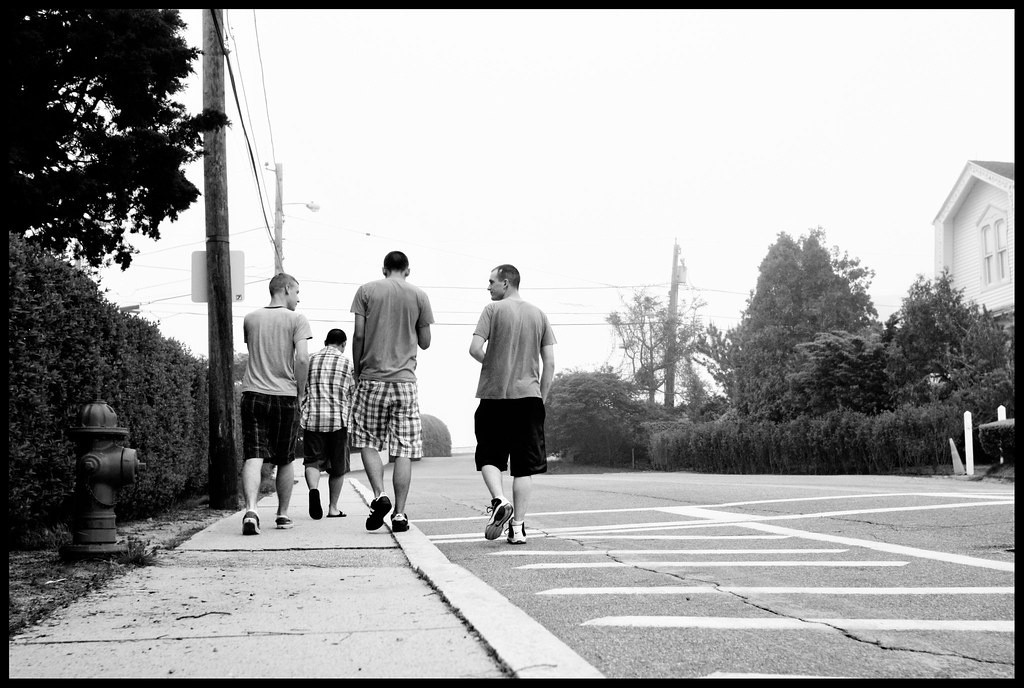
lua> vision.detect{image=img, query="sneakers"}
[504,517,526,544]
[366,492,392,530]
[391,513,408,532]
[485,496,514,540]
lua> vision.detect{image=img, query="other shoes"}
[242,511,261,535]
[275,515,293,529]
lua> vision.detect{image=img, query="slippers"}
[309,489,322,520]
[326,510,346,517]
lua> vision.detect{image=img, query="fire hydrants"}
[60,400,147,554]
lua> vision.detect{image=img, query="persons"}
[469,264,558,546]
[300,329,355,520]
[349,251,435,532]
[240,272,314,536]
